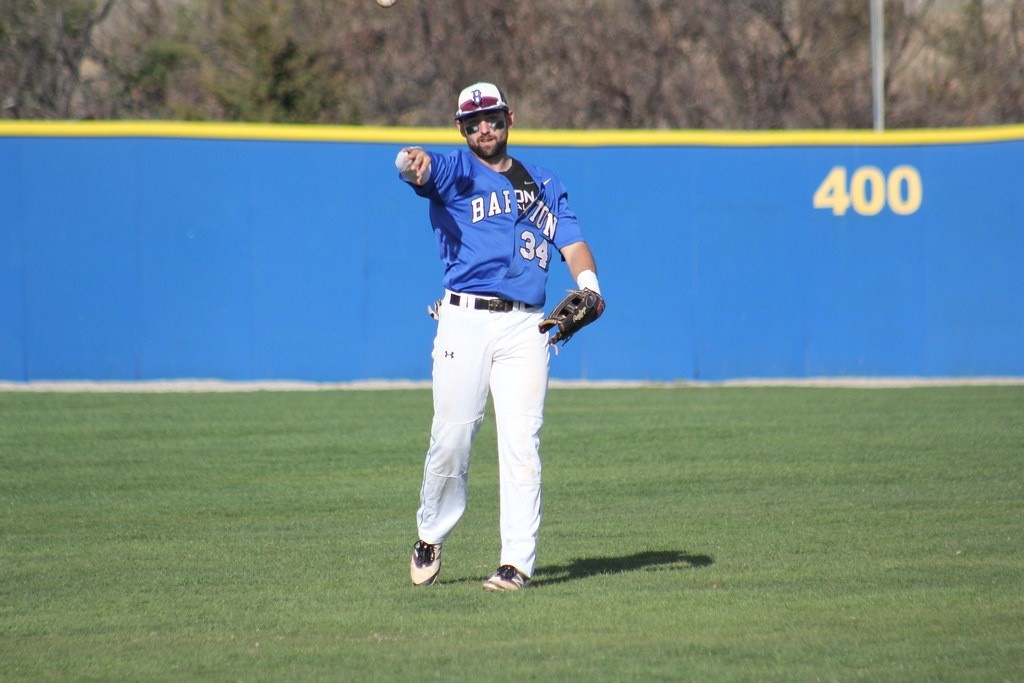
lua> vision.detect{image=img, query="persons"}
[395,82,606,592]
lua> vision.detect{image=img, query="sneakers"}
[409,540,442,587]
[482,563,527,593]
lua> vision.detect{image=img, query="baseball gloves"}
[537,289,606,347]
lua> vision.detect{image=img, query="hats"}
[454,80,509,119]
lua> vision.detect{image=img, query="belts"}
[449,294,533,313]
